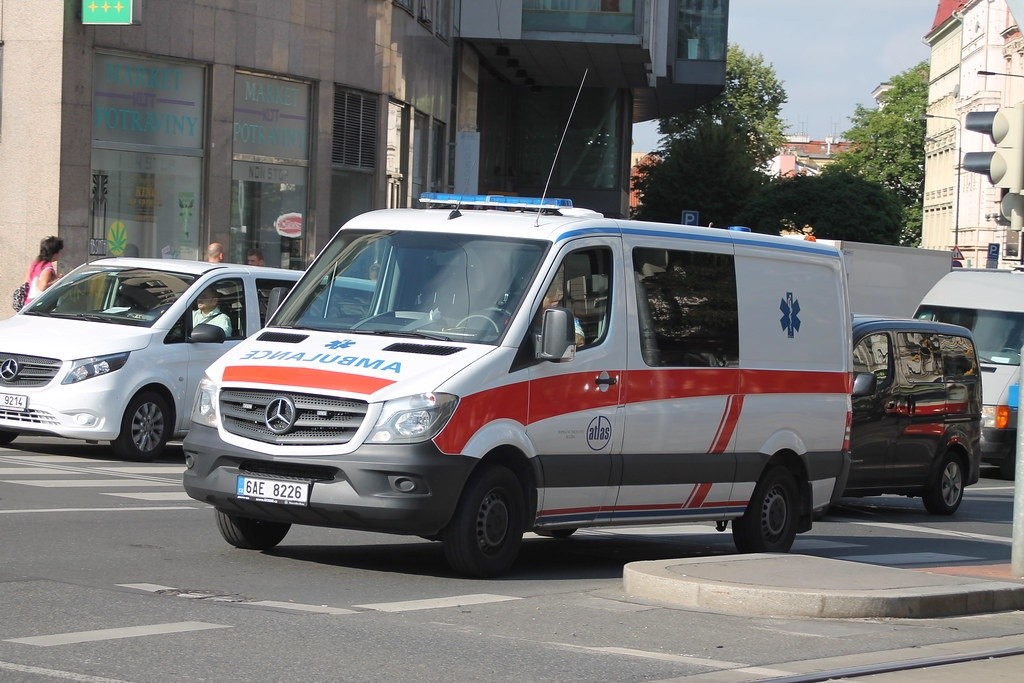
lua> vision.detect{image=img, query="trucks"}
[775,233,953,318]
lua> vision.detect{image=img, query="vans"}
[0,257,378,463]
[812,320,982,516]
[913,266,1024,482]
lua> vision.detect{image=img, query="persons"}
[542,271,585,349]
[207,243,226,263]
[192,282,232,338]
[23,236,64,315]
[247,247,267,267]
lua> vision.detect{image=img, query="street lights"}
[916,112,965,252]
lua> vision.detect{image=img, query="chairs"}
[403,259,487,318]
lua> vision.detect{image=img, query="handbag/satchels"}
[12,282,30,312]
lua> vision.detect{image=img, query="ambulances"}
[182,192,848,580]
